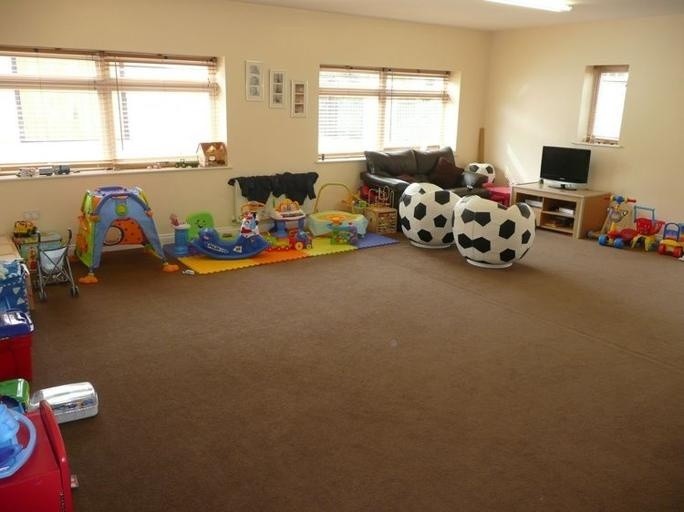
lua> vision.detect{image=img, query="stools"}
[486,186,511,208]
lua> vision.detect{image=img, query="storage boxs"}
[0,310,35,381]
[0,379,30,422]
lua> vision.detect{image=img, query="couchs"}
[359,147,488,233]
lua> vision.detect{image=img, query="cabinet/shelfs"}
[509,181,613,241]
[0,400,74,512]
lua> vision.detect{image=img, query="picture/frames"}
[269,71,285,108]
[290,80,309,119]
[245,59,264,101]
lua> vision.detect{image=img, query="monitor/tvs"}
[539,145,592,190]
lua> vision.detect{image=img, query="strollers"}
[31,228,80,301]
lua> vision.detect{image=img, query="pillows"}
[362,149,418,177]
[429,156,464,189]
[414,147,456,176]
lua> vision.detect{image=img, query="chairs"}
[451,195,536,269]
[399,182,462,249]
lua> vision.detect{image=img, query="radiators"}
[228,172,319,224]
